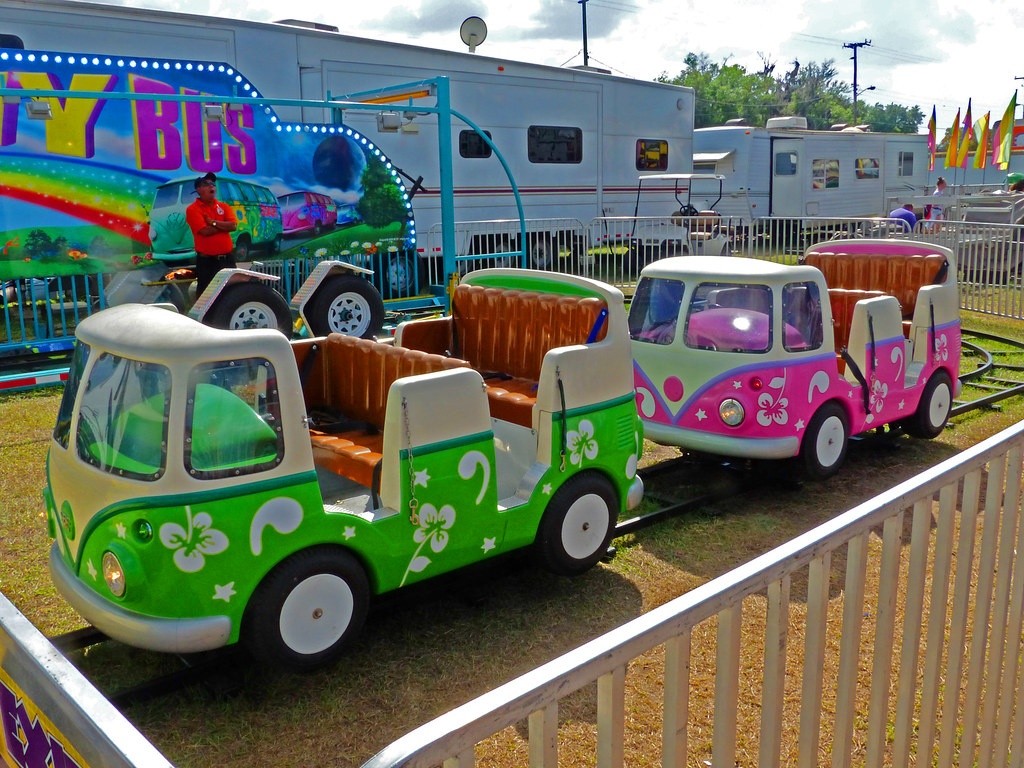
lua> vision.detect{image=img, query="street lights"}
[853,86,876,126]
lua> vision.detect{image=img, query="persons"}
[925,177,946,234]
[1009,179,1024,192]
[888,204,916,233]
[187,172,237,299]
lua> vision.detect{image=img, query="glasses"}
[201,181,216,188]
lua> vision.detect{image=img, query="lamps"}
[377,111,420,135]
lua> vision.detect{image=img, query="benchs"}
[781,252,946,350]
[270,283,609,491]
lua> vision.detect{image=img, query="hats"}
[195,172,216,189]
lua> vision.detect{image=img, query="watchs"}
[212,220,216,227]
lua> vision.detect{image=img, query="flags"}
[927,92,1016,171]
[812,157,879,189]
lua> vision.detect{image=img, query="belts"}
[199,253,232,259]
